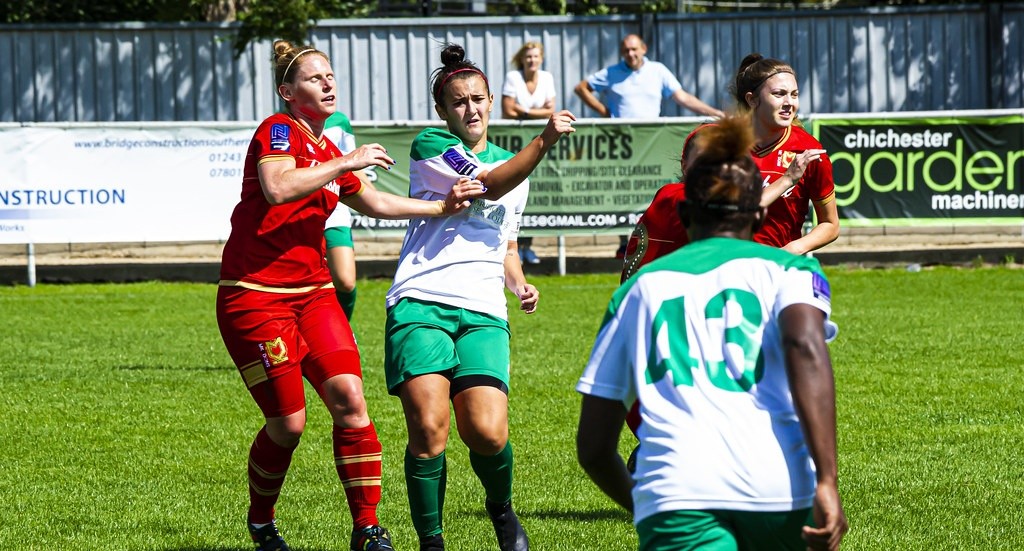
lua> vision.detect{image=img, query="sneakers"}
[524,248,540,264]
[518,248,524,265]
[349,527,396,550]
[247,518,290,551]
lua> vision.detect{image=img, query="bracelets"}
[524,110,529,118]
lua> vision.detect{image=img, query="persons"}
[215,39,489,551]
[731,54,839,255]
[575,35,725,259]
[384,44,576,551]
[500,43,556,264]
[571,104,849,551]
[322,111,377,323]
[619,121,722,283]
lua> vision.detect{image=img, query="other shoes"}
[419,533,445,551]
[616,244,628,258]
[486,499,529,551]
